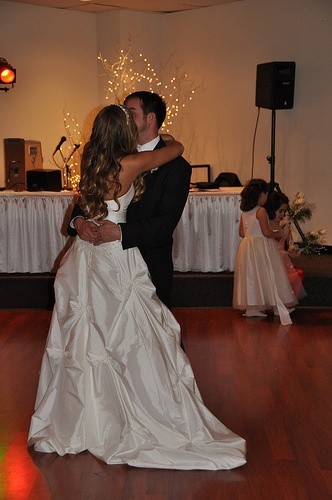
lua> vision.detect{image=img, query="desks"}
[0,188,242,274]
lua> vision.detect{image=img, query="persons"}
[264,191,307,315]
[53,97,183,466]
[233,179,299,317]
[67,91,192,352]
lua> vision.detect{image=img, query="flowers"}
[297,229,328,255]
[284,191,314,225]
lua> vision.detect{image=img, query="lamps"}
[0,57,17,92]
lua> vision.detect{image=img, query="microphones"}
[53,136,66,154]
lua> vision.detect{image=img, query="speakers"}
[255,61,296,110]
[27,169,62,192]
[4,138,43,191]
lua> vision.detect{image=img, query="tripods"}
[265,109,307,243]
